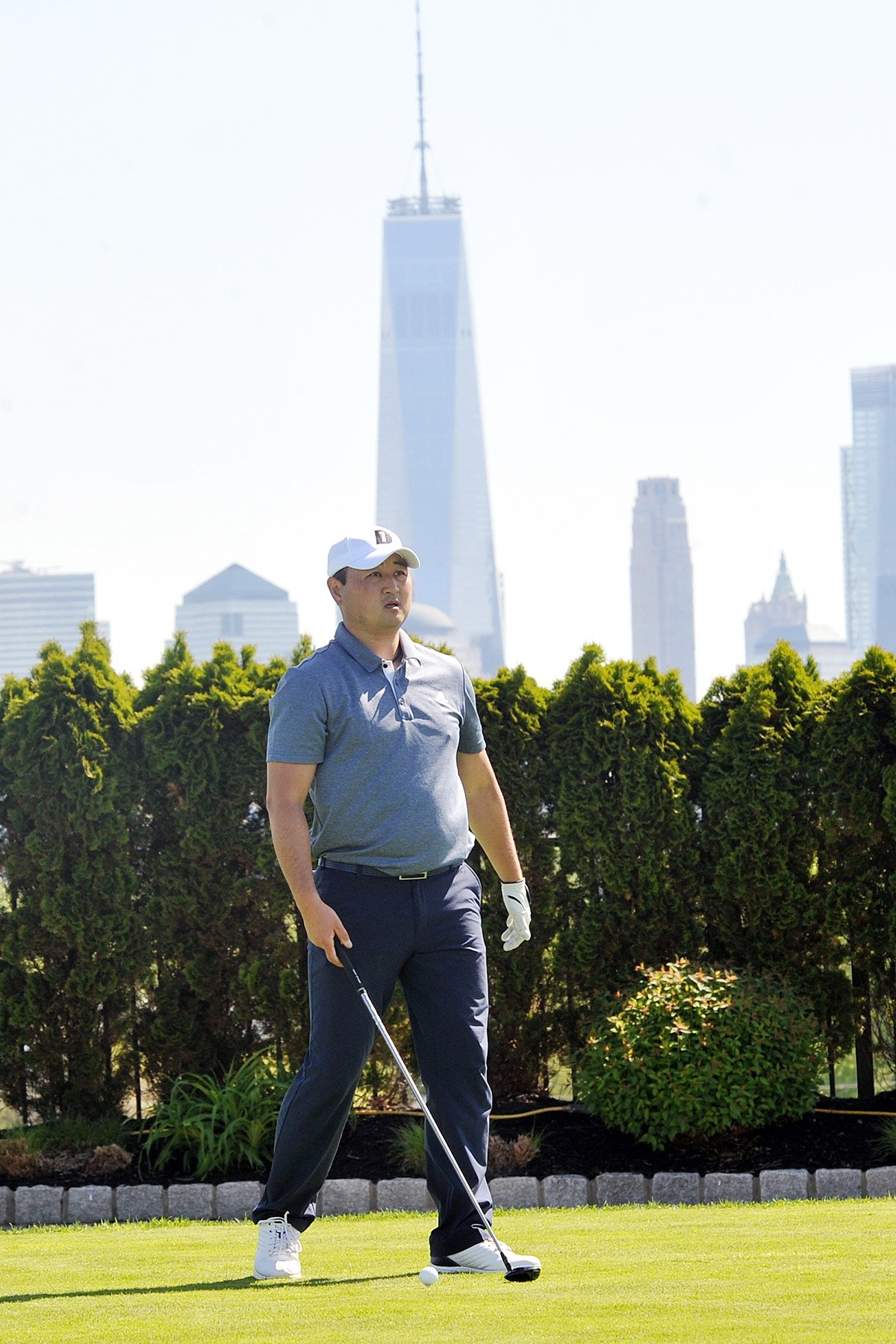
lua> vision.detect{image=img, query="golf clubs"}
[332,938,543,1284]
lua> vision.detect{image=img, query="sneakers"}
[252,1213,303,1280]
[429,1231,543,1279]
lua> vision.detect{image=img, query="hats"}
[326,525,422,577]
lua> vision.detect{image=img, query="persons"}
[252,526,540,1280]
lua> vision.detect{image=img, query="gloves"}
[495,878,531,953]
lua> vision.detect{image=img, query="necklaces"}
[386,647,401,663]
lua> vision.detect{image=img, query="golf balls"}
[420,1266,439,1286]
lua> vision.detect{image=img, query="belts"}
[318,860,465,881]
